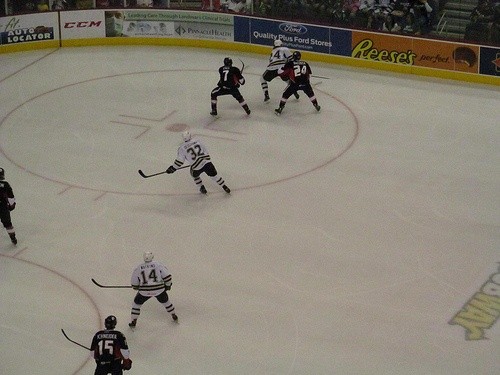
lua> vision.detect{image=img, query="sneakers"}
[171,313,179,323]
[7,232,18,247]
[274,104,285,116]
[129,318,137,331]
[315,105,322,113]
[222,185,230,193]
[200,184,207,193]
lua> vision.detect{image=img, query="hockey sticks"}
[239,58,244,74]
[138,165,190,179]
[91,278,132,288]
[61,328,90,350]
[311,75,332,79]
[311,81,322,85]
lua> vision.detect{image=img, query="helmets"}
[0,167,5,179]
[293,50,301,60]
[223,56,233,66]
[105,314,118,329]
[182,130,191,141]
[143,250,154,261]
[274,39,283,46]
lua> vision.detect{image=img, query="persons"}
[260,40,301,104]
[91,315,132,375]
[209,57,251,116]
[166,131,232,195]
[0,168,17,245]
[464,0,500,45]
[128,252,180,328]
[0,0,137,18]
[203,0,444,37]
[274,51,321,115]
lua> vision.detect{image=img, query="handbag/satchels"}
[391,9,404,18]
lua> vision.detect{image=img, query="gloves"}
[165,285,171,290]
[7,201,16,211]
[166,165,176,174]
[122,358,132,370]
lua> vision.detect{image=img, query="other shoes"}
[263,93,270,104]
[209,110,217,119]
[242,102,250,114]
[294,92,300,101]
